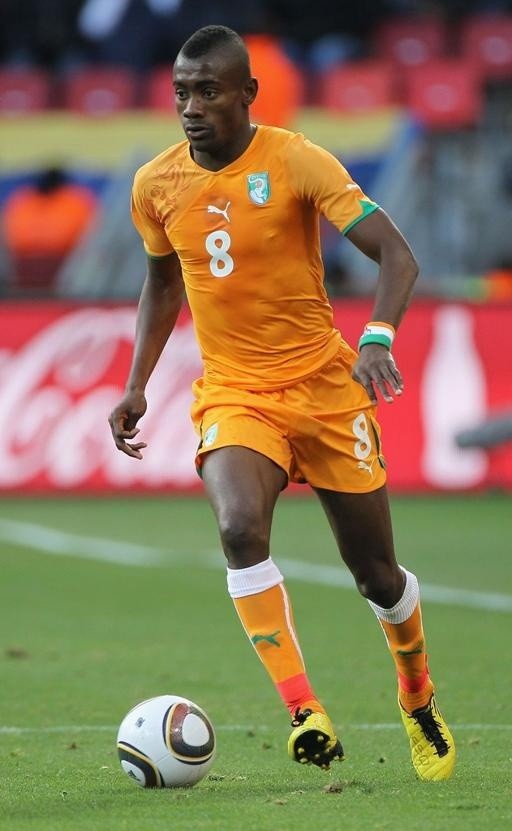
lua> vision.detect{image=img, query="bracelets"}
[354,321,397,352]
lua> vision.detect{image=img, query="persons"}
[0,0,512,300]
[109,23,461,786]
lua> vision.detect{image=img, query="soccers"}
[117,695,217,788]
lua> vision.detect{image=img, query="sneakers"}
[397,685,456,784]
[287,705,346,771]
